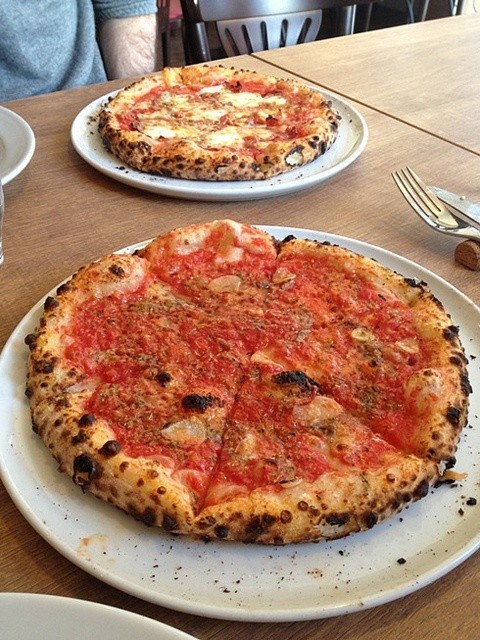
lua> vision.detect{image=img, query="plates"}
[70,84,369,203]
[0,223,480,624]
[0,591,201,640]
[0,106,37,187]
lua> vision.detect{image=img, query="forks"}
[391,165,480,243]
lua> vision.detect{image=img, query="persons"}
[2,1,160,102]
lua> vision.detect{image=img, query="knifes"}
[425,185,480,225]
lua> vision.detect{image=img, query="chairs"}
[180,1,356,65]
[155,0,171,68]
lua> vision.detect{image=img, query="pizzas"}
[25,217,472,543]
[98,63,342,180]
